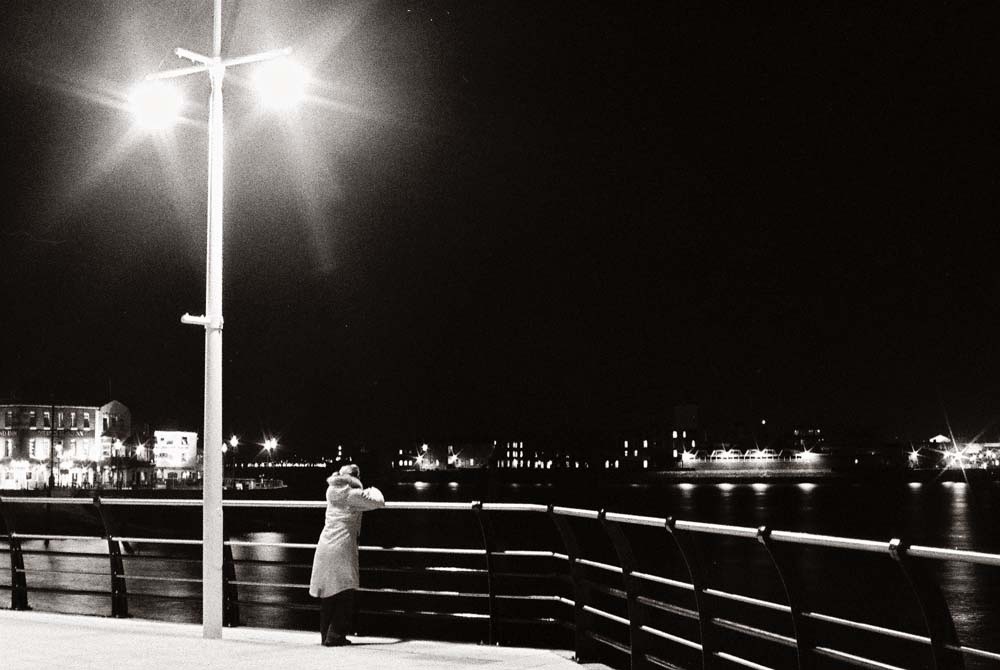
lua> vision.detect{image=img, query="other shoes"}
[322,639,353,646]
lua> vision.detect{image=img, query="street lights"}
[265,438,278,479]
[132,0,311,641]
[223,436,239,487]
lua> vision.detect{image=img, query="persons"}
[309,463,385,647]
